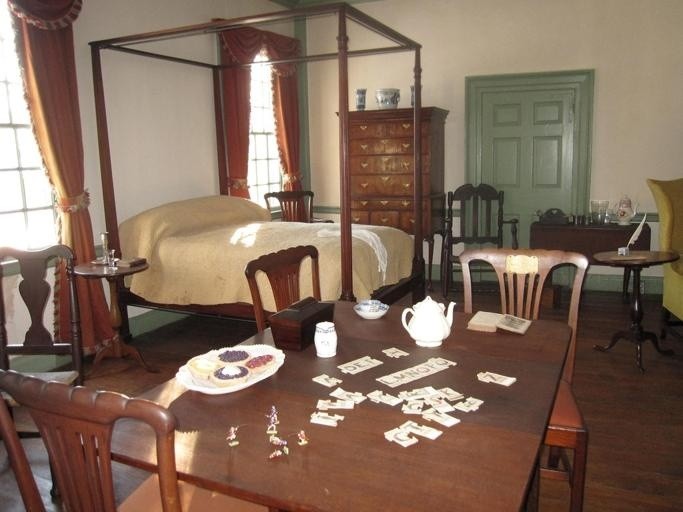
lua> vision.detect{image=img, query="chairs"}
[0,244,82,498]
[440,181,518,297]
[245,245,321,332]
[0,368,278,512]
[646,176,683,349]
[265,190,334,223]
[458,247,591,512]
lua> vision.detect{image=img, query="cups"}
[573,215,590,225]
[104,249,115,267]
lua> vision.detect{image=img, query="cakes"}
[189,350,275,387]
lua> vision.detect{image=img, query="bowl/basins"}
[353,299,389,320]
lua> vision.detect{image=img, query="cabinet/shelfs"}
[335,106,450,239]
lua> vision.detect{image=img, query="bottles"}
[591,200,609,225]
[313,322,337,358]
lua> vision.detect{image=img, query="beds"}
[88,2,422,336]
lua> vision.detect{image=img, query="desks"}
[74,258,161,373]
[531,220,651,305]
[593,249,683,375]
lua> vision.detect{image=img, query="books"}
[466,311,532,335]
[115,257,146,268]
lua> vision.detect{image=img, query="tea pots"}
[401,294,457,347]
[612,194,640,226]
